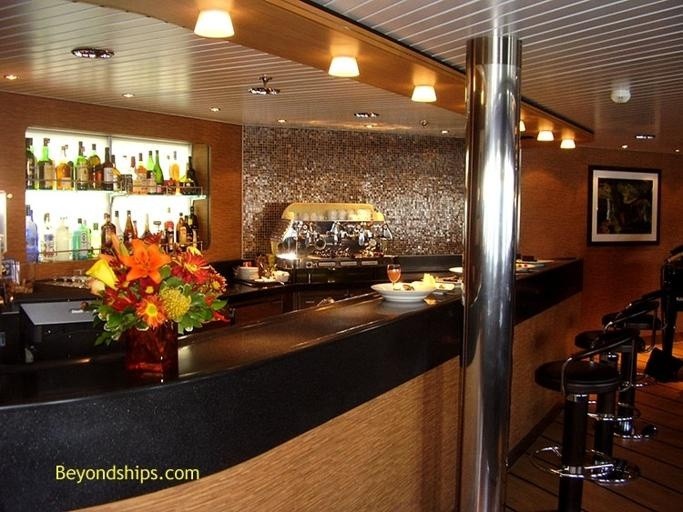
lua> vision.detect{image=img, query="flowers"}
[86,226,231,347]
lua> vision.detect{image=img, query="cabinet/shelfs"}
[2,91,243,292]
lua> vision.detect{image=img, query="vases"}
[120,315,179,385]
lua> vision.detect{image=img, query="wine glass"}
[387,263,401,291]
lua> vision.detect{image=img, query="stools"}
[530,288,665,511]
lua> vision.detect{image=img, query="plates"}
[412,280,455,296]
[515,263,537,273]
[449,266,464,277]
[370,282,436,302]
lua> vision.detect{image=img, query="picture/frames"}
[587,166,662,246]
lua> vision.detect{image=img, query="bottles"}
[25,136,202,264]
